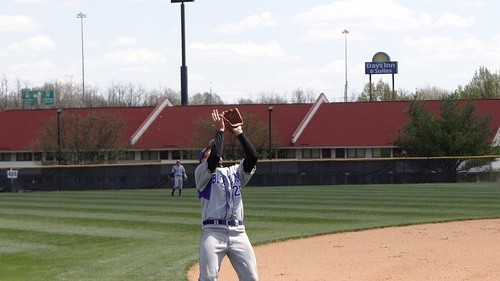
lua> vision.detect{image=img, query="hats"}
[176,159,181,162]
[199,138,218,163]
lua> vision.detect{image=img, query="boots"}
[178,189,182,197]
[171,188,176,197]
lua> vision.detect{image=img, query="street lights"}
[342,29,349,102]
[77,12,87,108]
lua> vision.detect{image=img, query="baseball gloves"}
[218,108,243,133]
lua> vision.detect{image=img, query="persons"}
[194,108,258,281]
[167,159,188,196]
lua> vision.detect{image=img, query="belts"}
[203,218,243,226]
